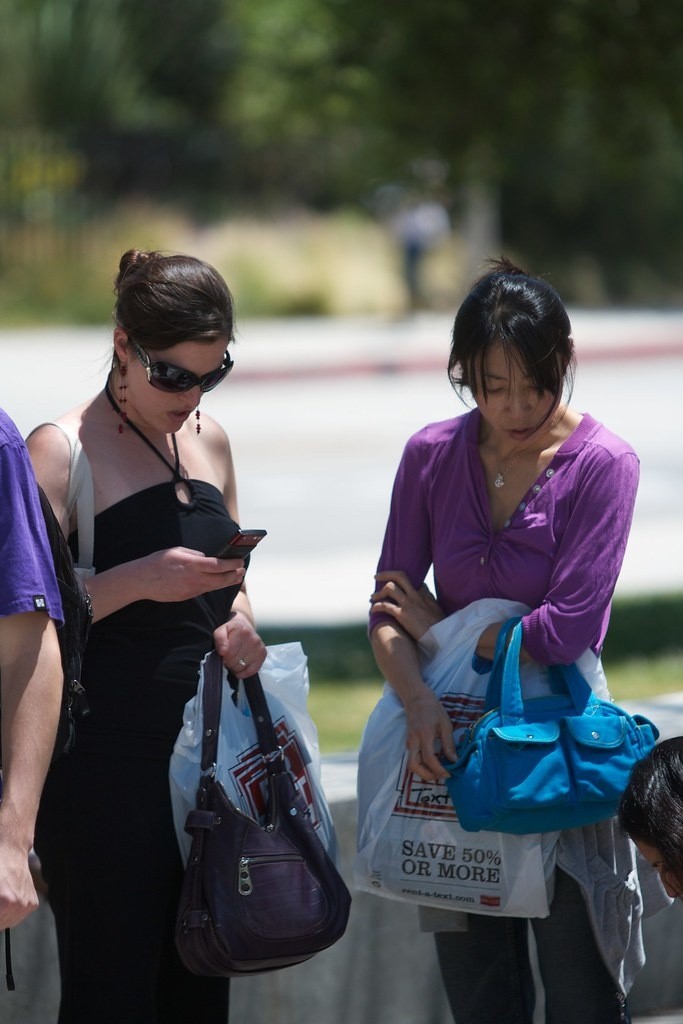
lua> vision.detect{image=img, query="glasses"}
[128,335,233,392]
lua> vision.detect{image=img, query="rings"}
[239,659,248,668]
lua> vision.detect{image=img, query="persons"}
[0,409,67,934]
[21,250,268,1024]
[617,734,683,902]
[367,265,645,1024]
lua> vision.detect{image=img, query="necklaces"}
[494,417,554,489]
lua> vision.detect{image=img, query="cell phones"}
[217,530,268,560]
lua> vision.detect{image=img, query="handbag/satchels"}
[169,642,340,884]
[439,617,659,834]
[175,652,353,977]
[353,599,609,919]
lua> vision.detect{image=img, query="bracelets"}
[84,592,94,617]
